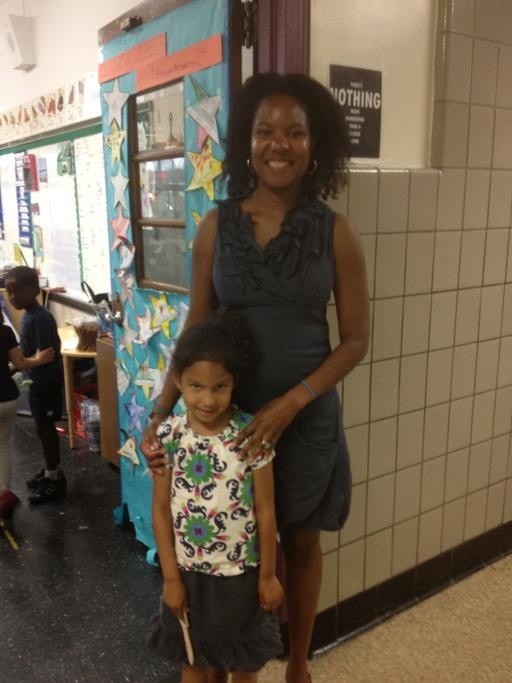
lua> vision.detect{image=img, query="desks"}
[55,325,97,450]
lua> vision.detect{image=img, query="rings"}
[262,439,271,449]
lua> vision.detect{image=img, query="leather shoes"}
[0,490,20,518]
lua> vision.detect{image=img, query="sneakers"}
[25,469,44,488]
[28,469,67,503]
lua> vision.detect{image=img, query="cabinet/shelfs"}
[95,335,118,468]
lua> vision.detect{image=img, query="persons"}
[0,303,56,511]
[147,320,285,683]
[140,74,371,683]
[4,267,67,503]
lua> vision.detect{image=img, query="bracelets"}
[300,380,317,400]
[150,405,173,418]
